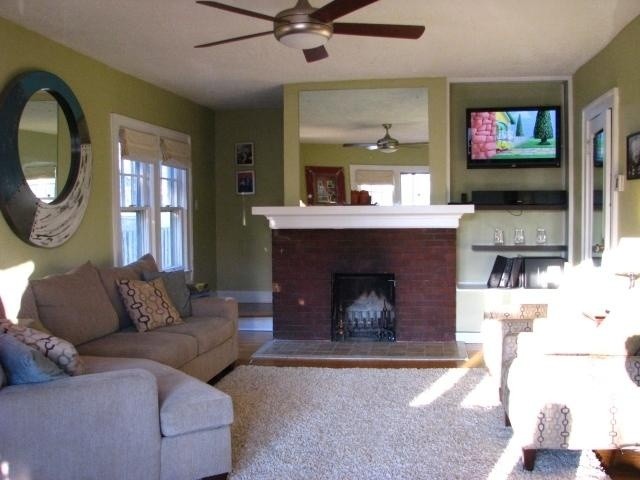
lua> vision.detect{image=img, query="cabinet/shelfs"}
[448,204,569,341]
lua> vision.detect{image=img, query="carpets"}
[214,364,613,480]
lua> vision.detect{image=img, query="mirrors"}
[298,86,432,206]
[0,70,92,249]
[586,109,611,268]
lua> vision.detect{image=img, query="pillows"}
[143,270,193,318]
[0,333,71,385]
[114,276,185,333]
[0,318,87,376]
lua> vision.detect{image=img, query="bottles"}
[350,190,369,204]
[535,224,547,244]
[494,224,504,244]
[512,224,525,245]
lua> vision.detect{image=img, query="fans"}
[194,0,425,63]
[342,124,430,153]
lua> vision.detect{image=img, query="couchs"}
[0,254,239,480]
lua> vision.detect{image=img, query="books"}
[486,255,523,289]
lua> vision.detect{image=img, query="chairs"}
[483,303,547,376]
[500,282,640,471]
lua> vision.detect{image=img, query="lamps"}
[614,237,640,289]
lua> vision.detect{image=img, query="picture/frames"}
[626,131,640,180]
[235,142,254,166]
[306,166,346,206]
[235,170,255,195]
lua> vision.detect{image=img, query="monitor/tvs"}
[465,105,562,170]
[592,128,604,168]
